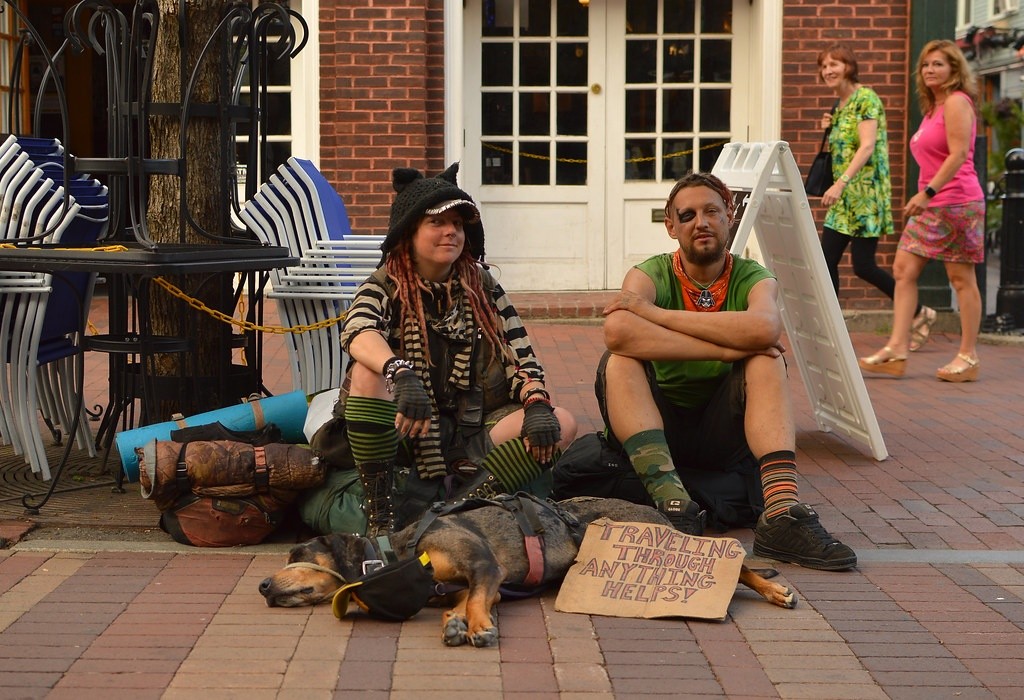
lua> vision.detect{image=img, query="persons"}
[594,172,859,571]
[817,41,937,353]
[340,163,577,539]
[858,39,986,383]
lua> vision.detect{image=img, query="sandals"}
[910,305,937,352]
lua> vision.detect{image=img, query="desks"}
[0,0,310,261]
[0,256,300,516]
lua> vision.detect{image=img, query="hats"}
[377,161,490,270]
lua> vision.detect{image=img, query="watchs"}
[841,174,849,182]
[924,185,936,198]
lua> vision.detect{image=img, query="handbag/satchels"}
[298,463,418,536]
[158,423,315,547]
[804,98,840,198]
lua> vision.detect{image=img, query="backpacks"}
[552,431,766,534]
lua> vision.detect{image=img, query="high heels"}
[936,353,980,381]
[859,346,908,376]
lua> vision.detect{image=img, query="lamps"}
[963,26,1024,51]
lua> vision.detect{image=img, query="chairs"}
[0,131,109,477]
[239,158,389,399]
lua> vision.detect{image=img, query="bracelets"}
[383,357,414,393]
[521,387,551,408]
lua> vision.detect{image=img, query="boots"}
[449,466,505,506]
[354,458,398,540]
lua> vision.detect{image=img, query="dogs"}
[259,496,796,647]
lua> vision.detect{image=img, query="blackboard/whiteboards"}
[710,140,883,445]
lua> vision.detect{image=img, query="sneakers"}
[658,499,707,538]
[753,503,856,570]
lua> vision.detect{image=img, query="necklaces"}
[683,265,726,308]
